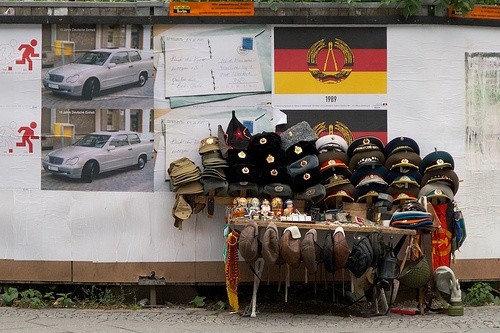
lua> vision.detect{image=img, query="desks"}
[233,218,417,319]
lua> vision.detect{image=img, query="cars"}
[42,47,156,102]
[42,129,155,185]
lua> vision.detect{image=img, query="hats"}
[420,168,459,195]
[418,147,454,173]
[261,222,279,285]
[301,228,318,284]
[279,225,301,302]
[350,231,374,294]
[332,226,349,297]
[167,110,421,229]
[323,230,334,275]
[418,182,454,204]
[238,222,258,263]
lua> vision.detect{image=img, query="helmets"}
[397,257,431,289]
